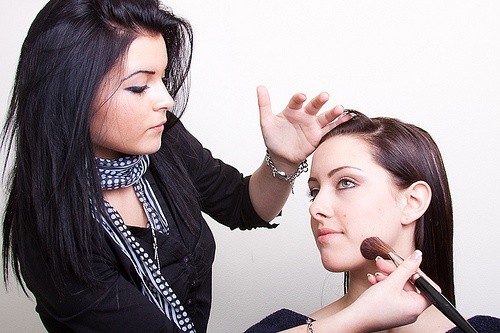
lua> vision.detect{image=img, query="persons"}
[1,0,444,333]
[229,105,500,333]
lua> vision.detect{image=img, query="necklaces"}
[143,208,163,277]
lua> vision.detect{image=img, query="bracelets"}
[305,316,316,333]
[265,150,311,196]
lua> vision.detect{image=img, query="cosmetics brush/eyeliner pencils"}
[359,234,479,333]
